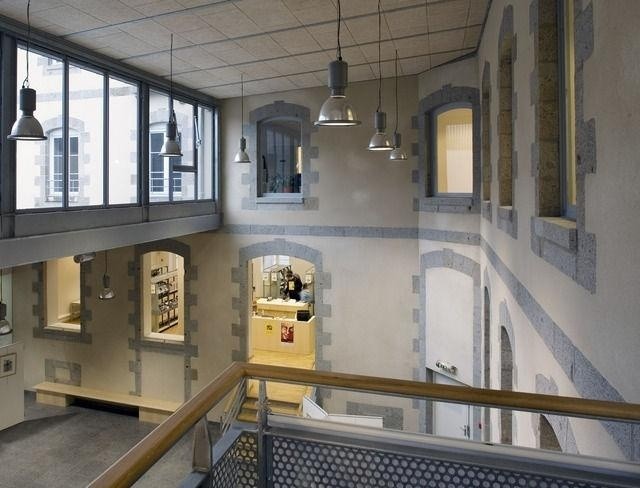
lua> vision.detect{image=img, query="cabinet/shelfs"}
[263,264,292,298]
[151,265,178,332]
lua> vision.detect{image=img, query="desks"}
[256,297,311,319]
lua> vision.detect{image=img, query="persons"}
[298,282,314,304]
[287,270,300,279]
[281,324,289,342]
[283,272,301,300]
[287,326,294,343]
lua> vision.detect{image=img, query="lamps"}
[7,1,48,141]
[158,34,184,156]
[232,72,254,163]
[98,249,117,300]
[0,268,15,335]
[312,1,408,162]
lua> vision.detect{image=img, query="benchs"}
[31,381,183,425]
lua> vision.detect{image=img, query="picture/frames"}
[0,352,17,378]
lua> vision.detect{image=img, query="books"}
[288,281,295,290]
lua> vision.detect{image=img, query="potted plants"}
[283,175,294,192]
